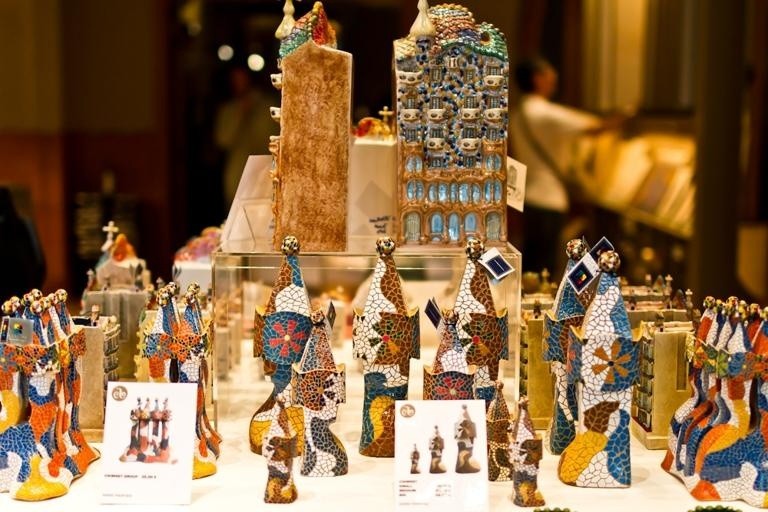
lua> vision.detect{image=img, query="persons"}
[216,59,277,227]
[509,58,630,282]
